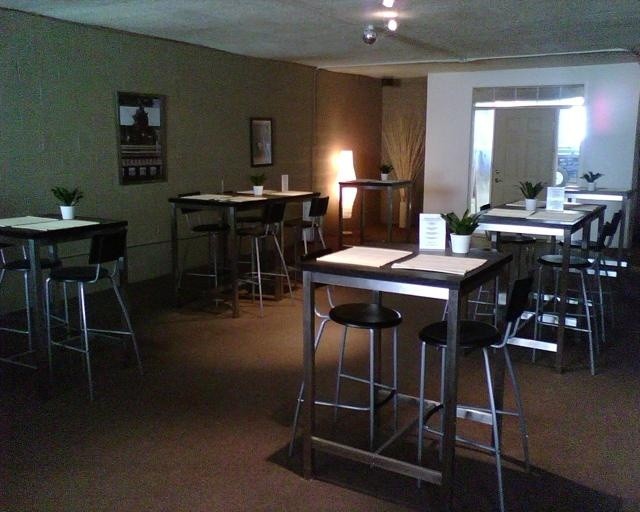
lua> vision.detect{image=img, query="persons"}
[255,141,265,162]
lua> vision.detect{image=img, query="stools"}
[45,219,142,398]
[0,237,63,352]
[442,232,500,330]
[286,196,328,255]
[532,209,624,376]
[235,197,293,318]
[177,190,234,289]
[290,247,403,457]
[417,271,534,511]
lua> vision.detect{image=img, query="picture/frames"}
[249,116,273,167]
[115,89,168,186]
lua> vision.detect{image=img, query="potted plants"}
[579,173,604,191]
[51,185,85,220]
[438,209,482,254]
[379,164,392,177]
[251,174,265,197]
[379,110,426,229]
[512,180,547,211]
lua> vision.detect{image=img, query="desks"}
[295,247,515,508]
[474,200,608,376]
[565,187,636,259]
[169,189,321,317]
[338,179,414,251]
[1,213,130,385]
